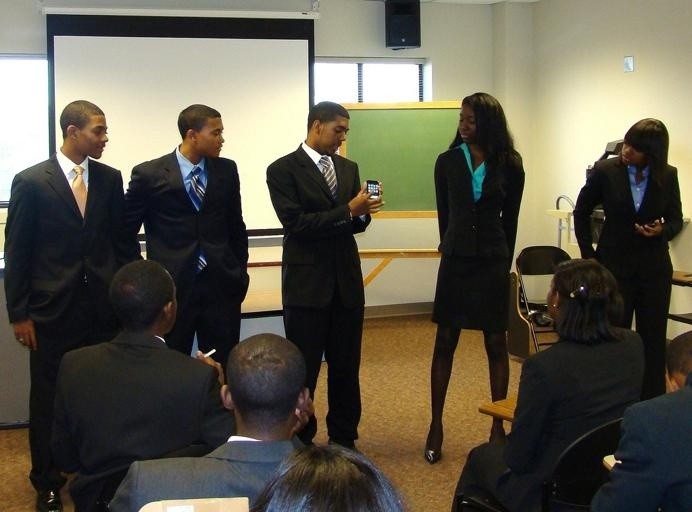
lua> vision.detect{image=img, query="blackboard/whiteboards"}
[339,101,464,219]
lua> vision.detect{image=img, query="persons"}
[570,119,684,399]
[265,100,386,456]
[97,329,404,512]
[123,104,252,367]
[584,330,691,512]
[449,256,647,511]
[240,444,386,512]
[45,258,233,512]
[5,100,153,511]
[422,91,524,463]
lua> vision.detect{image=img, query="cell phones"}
[367,180,379,198]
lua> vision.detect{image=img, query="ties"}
[319,157,337,201]
[72,166,88,219]
[189,167,208,271]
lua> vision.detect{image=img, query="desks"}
[477,396,519,442]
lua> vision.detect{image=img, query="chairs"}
[515,244,572,354]
[456,419,622,509]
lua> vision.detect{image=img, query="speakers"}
[385,0,421,50]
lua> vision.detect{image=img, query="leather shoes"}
[37,486,64,512]
[424,446,441,464]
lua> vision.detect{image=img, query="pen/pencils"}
[204,348,217,357]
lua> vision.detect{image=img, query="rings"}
[19,339,24,344]
